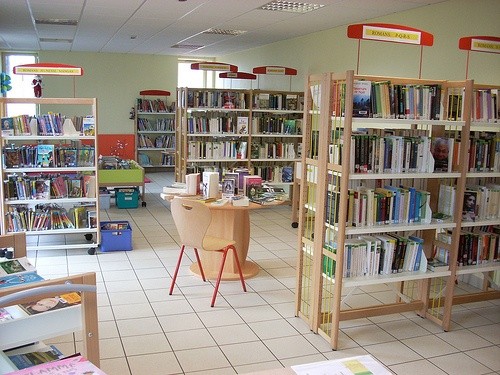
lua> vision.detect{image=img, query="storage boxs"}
[99,188,112,210]
[100,220,132,252]
[114,187,140,209]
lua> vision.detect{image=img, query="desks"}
[171,184,288,281]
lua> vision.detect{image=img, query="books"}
[252,94,304,111]
[250,141,304,158]
[97,155,135,169]
[461,183,499,221]
[2,172,97,200]
[160,165,293,206]
[1,112,96,136]
[472,90,500,122]
[2,143,95,168]
[329,132,455,176]
[137,98,175,112]
[324,232,453,278]
[327,81,467,121]
[101,220,129,235]
[187,139,247,160]
[0,256,37,278]
[452,142,499,172]
[138,118,174,131]
[182,115,248,134]
[0,272,45,290]
[252,115,302,135]
[136,134,175,147]
[4,341,108,375]
[137,151,175,165]
[17,289,84,316]
[118,188,135,201]
[326,182,454,226]
[449,227,499,267]
[182,90,246,109]
[1,308,14,324]
[3,204,98,232]
[98,187,110,195]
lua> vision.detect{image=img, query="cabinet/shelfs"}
[295,69,500,353]
[1,97,100,244]
[174,84,304,192]
[137,96,173,171]
[0,231,119,375]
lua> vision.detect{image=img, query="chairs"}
[168,196,247,307]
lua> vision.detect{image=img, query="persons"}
[31,74,45,98]
[463,194,475,210]
[27,291,80,315]
[433,139,448,171]
